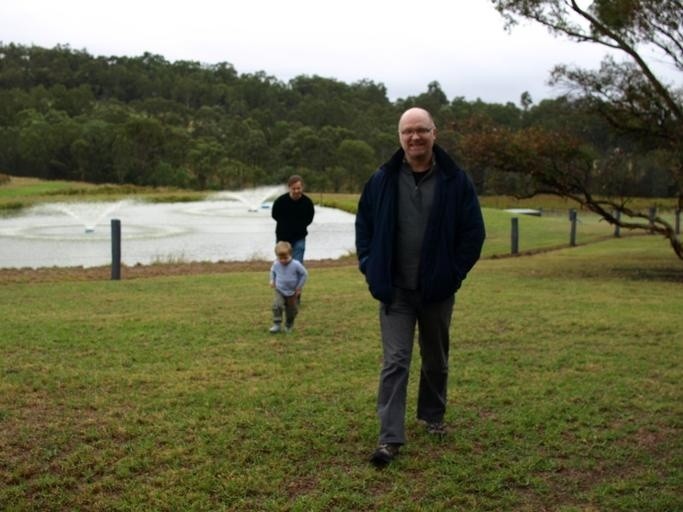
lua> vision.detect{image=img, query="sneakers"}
[370,442,401,467]
[269,324,292,333]
[423,419,446,434]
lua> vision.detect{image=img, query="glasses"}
[401,127,431,135]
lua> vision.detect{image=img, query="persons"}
[351,105,487,468]
[267,240,308,334]
[270,173,315,262]
